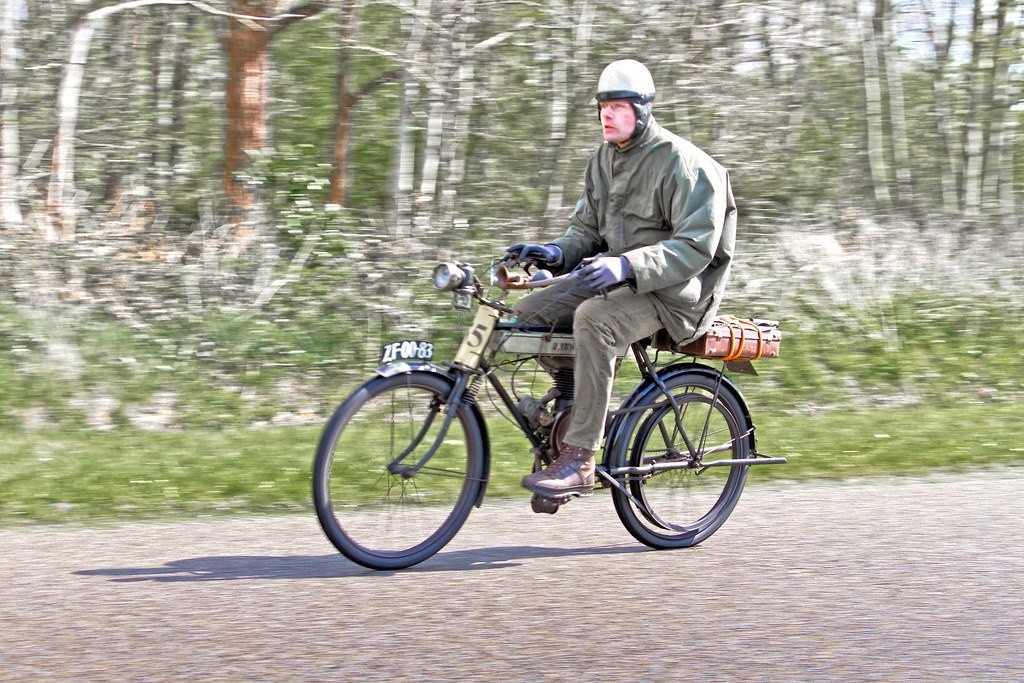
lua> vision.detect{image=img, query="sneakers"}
[522,445,595,499]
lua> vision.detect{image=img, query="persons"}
[506,56,736,496]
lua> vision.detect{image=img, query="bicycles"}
[313,251,787,570]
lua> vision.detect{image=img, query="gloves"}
[581,256,632,292]
[501,244,559,264]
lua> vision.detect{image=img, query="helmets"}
[595,59,656,105]
